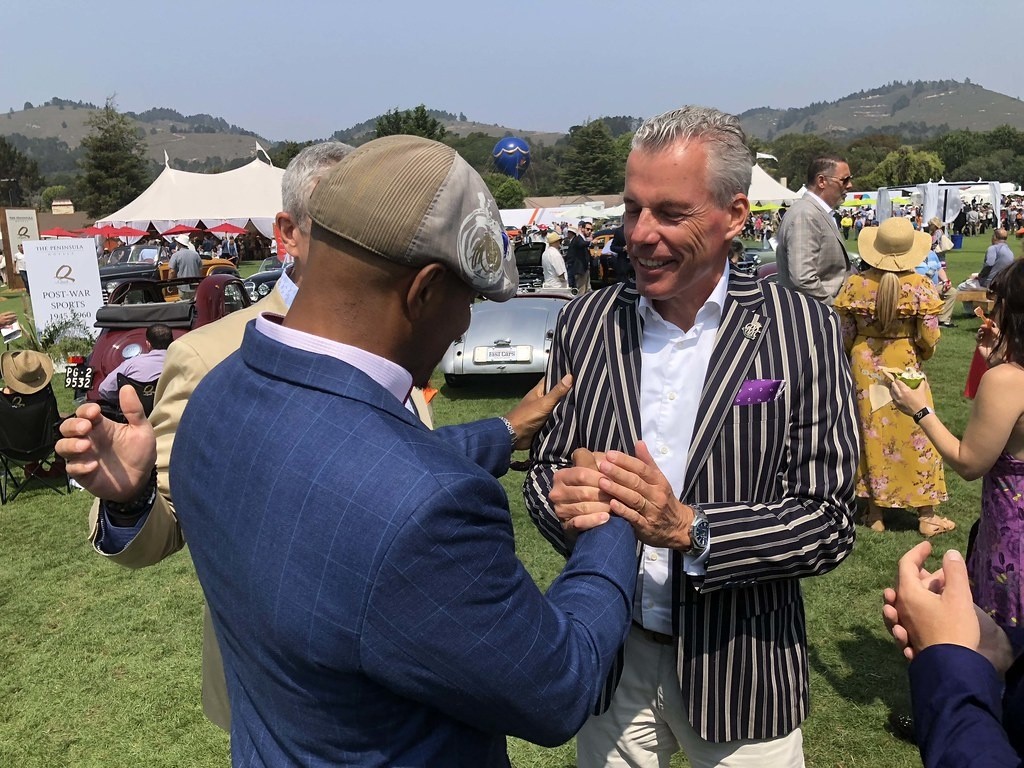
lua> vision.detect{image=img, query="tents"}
[606,202,625,222]
[95,158,287,246]
[559,204,608,222]
[746,162,799,205]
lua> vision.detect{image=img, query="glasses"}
[818,174,854,184]
[583,227,594,232]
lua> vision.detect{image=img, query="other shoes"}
[888,708,918,744]
[938,322,959,328]
[49,465,63,480]
[23,463,49,478]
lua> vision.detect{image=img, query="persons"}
[0,350,66,478]
[952,196,995,237]
[56,143,434,729]
[915,247,958,327]
[167,235,202,300]
[889,256,1024,630]
[958,228,1014,314]
[740,211,781,242]
[1000,193,1024,233]
[884,539,1024,768]
[167,133,636,768]
[774,153,853,310]
[833,218,956,538]
[834,205,878,240]
[97,324,175,406]
[893,203,923,231]
[141,235,276,268]
[522,107,859,768]
[928,216,946,272]
[0,244,30,296]
[0,311,18,329]
[512,218,637,295]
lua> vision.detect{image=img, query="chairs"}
[0,391,76,505]
[96,373,159,426]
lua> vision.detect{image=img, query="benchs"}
[956,291,994,311]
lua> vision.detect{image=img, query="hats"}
[307,133,520,303]
[174,234,189,246]
[931,217,943,229]
[567,227,577,235]
[0,349,55,395]
[546,232,562,244]
[858,217,932,271]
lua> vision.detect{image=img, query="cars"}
[75,240,291,424]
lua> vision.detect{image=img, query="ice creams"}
[900,366,924,378]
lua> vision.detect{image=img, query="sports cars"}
[441,227,619,389]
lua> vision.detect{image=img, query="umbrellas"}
[842,199,877,207]
[204,223,248,239]
[891,197,912,205]
[161,223,202,235]
[81,225,150,244]
[41,227,77,240]
[762,203,784,210]
[750,204,765,211]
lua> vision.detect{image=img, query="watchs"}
[682,503,710,556]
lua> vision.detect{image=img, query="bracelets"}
[105,464,158,515]
[912,406,934,423]
[500,414,519,451]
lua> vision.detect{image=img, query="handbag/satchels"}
[933,228,954,254]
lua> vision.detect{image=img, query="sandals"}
[919,515,955,535]
[860,512,886,533]
